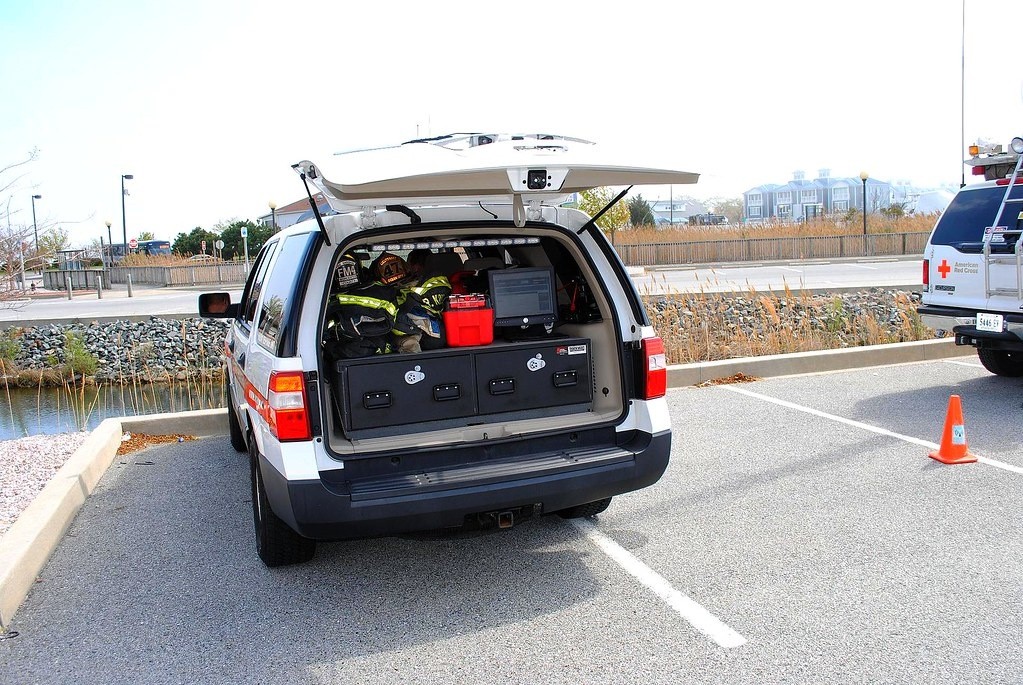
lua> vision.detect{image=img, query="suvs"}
[197,130,700,568]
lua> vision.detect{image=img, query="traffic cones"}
[927,394,975,464]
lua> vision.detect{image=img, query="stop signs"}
[129,238,138,248]
[201,241,206,250]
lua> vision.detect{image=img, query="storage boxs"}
[442,306,494,347]
[322,332,594,431]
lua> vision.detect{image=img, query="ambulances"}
[917,138,1022,374]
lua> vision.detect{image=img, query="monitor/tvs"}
[488,265,569,342]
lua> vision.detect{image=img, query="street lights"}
[106,222,113,264]
[268,201,279,236]
[120,172,134,259]
[31,194,43,277]
[860,170,870,258]
